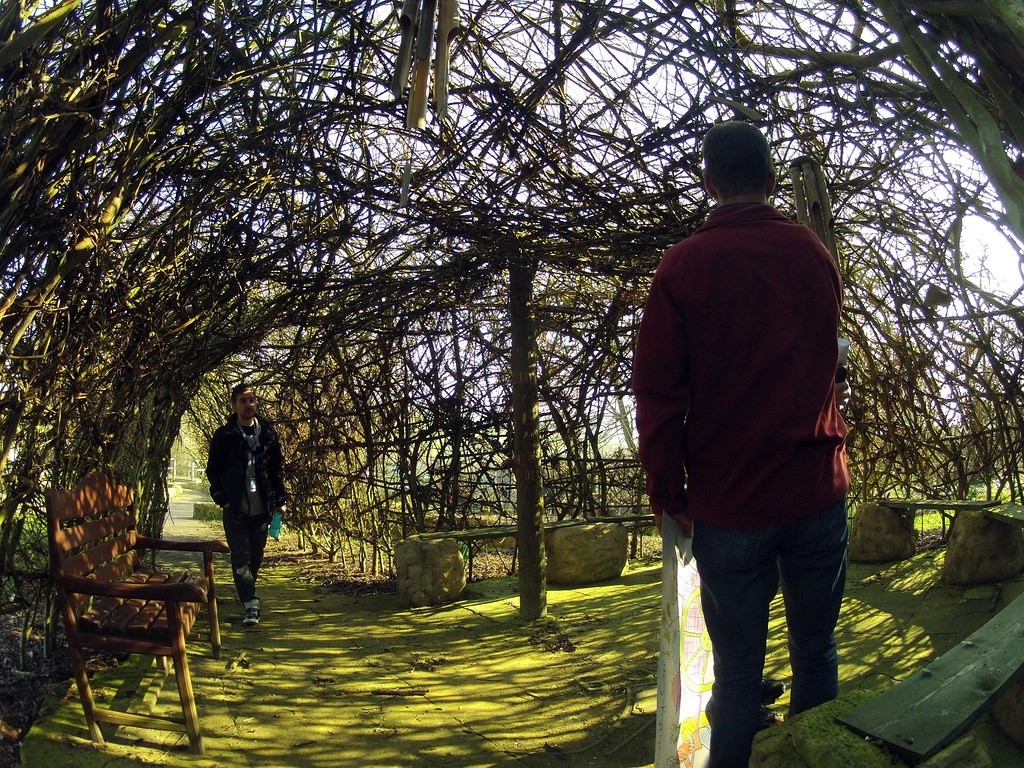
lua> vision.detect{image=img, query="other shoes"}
[760,706,784,730]
[760,678,782,705]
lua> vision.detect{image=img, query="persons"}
[629,118,853,768]
[205,382,288,624]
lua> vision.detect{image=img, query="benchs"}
[44,473,230,755]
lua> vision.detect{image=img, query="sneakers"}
[243,608,261,624]
